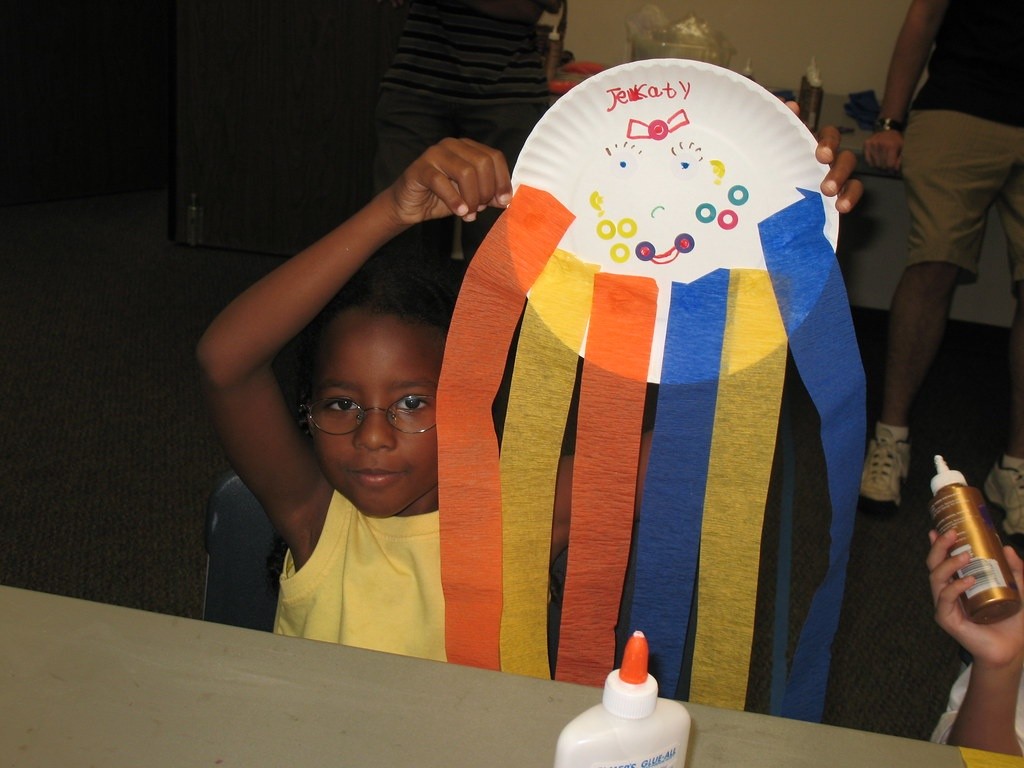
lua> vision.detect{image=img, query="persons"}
[865,0,1024,534]
[926,531,1024,757]
[196,101,863,663]
[375,0,563,323]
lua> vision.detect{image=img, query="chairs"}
[197,474,698,702]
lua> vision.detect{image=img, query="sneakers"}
[860,427,912,510]
[983,460,1024,538]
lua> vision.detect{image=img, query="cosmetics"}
[929,455,1022,624]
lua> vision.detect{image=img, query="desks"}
[547,67,1018,329]
[0,584,1024,768]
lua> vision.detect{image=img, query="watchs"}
[873,118,904,132]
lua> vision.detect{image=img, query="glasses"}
[299,394,438,436]
